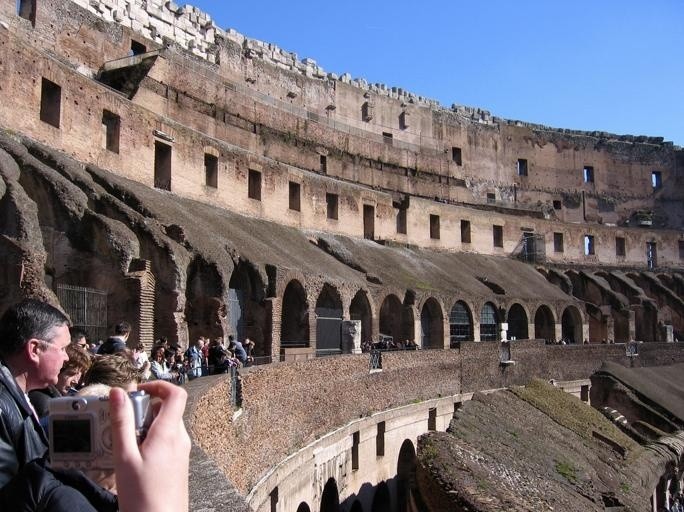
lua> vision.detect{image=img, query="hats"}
[244,339,249,344]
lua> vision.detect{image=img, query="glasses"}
[77,343,86,348]
[40,341,65,354]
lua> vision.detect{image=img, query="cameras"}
[48,391,152,471]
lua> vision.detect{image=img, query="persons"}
[545,338,567,345]
[148,335,254,387]
[25,321,152,423]
[1,298,72,487]
[601,338,607,344]
[0,380,193,512]
[675,338,678,342]
[584,338,589,345]
[609,337,613,344]
[360,340,419,369]
